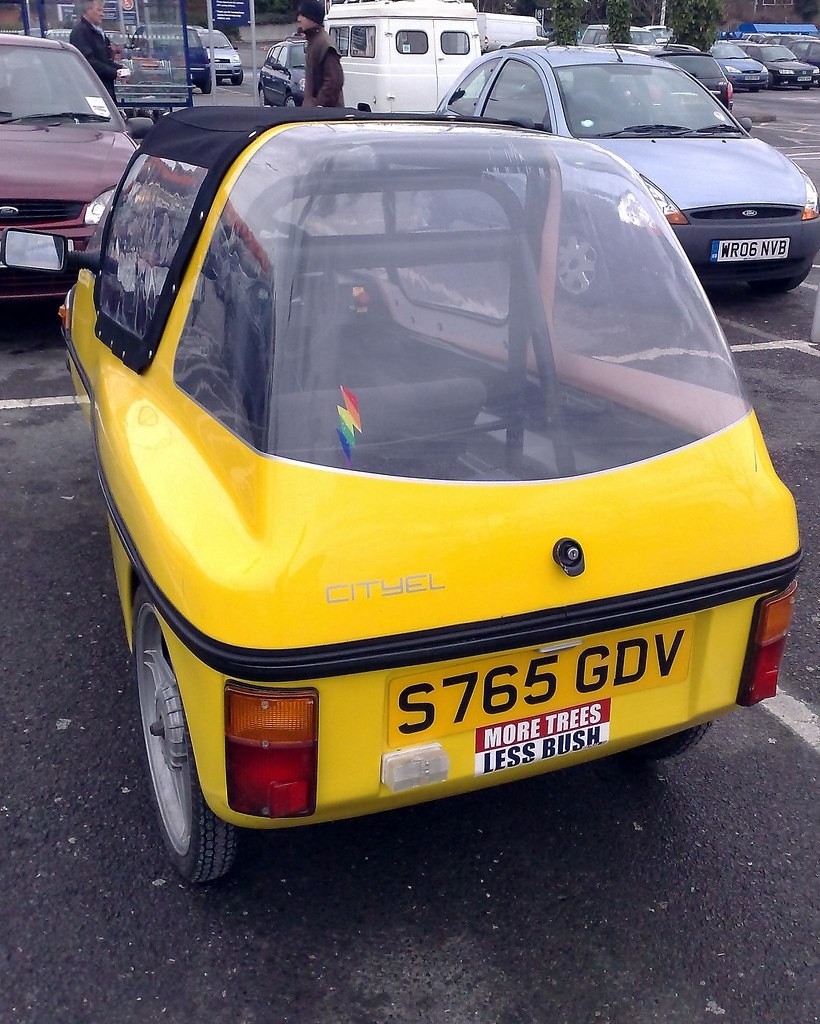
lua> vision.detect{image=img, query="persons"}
[297,0,345,108]
[70,0,132,107]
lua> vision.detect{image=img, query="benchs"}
[273,377,487,477]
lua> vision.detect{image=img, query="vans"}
[324,0,482,114]
[476,13,549,53]
[579,23,657,46]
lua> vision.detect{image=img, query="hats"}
[296,0,325,25]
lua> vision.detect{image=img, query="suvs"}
[590,36,733,119]
[121,24,212,94]
[194,26,244,86]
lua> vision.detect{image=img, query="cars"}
[642,25,678,43]
[258,40,308,108]
[707,33,820,92]
[0,33,155,300]
[434,49,818,302]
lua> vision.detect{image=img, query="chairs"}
[8,68,52,105]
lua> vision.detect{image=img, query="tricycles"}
[66,103,802,885]
